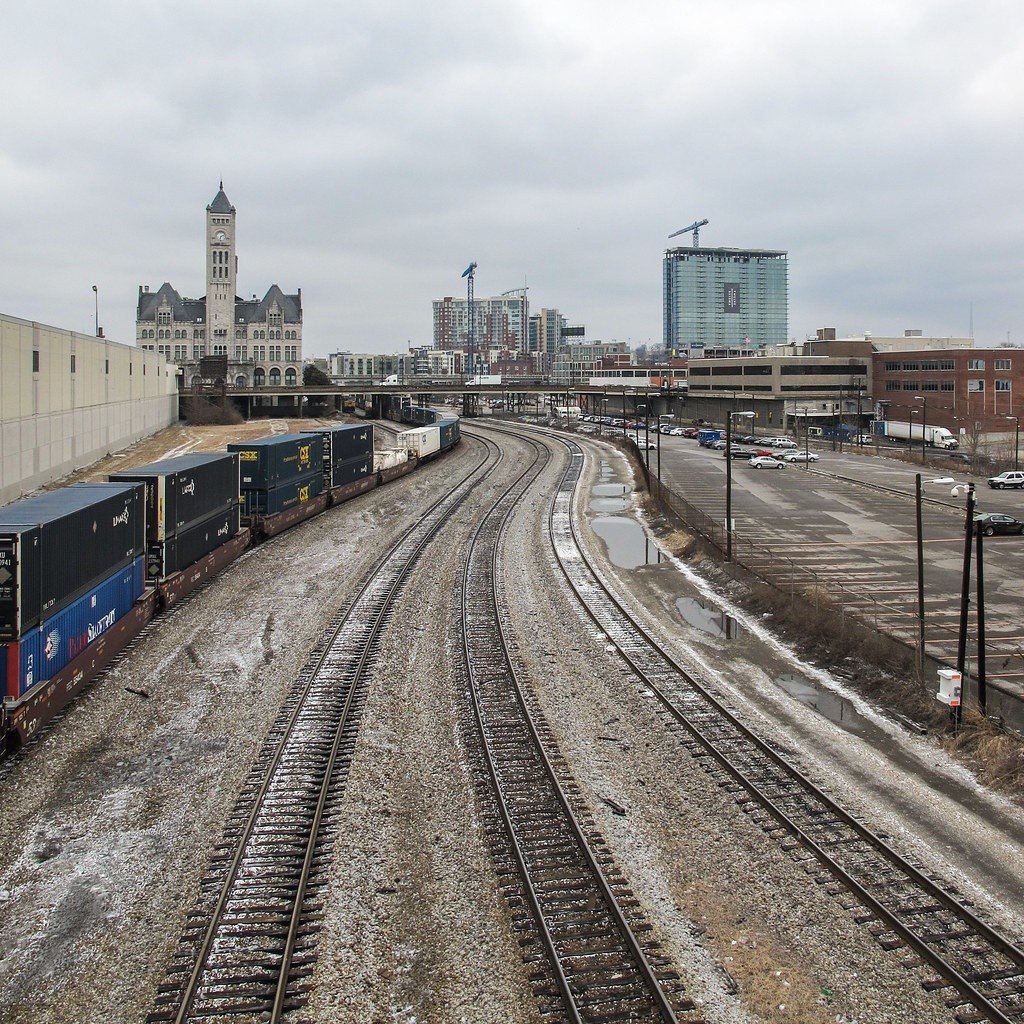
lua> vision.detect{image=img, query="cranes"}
[667,219,709,247]
[461,262,478,374]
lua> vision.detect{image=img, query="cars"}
[771,447,800,460]
[550,404,800,459]
[747,456,787,469]
[784,450,821,463]
[964,511,1024,537]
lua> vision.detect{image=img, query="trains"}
[0,399,462,759]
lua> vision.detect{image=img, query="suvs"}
[986,471,1024,489]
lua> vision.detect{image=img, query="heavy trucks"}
[884,420,960,451]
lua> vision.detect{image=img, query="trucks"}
[379,375,399,387]
[464,374,501,388]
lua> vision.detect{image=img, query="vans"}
[852,434,873,445]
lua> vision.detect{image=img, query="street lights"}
[796,406,809,469]
[599,397,609,435]
[92,284,100,337]
[643,391,663,493]
[622,387,637,445]
[721,411,760,561]
[909,409,919,454]
[656,413,676,500]
[826,402,836,451]
[914,394,927,461]
[913,473,956,688]
[567,386,575,428]
[1005,415,1019,471]
[634,404,646,451]
